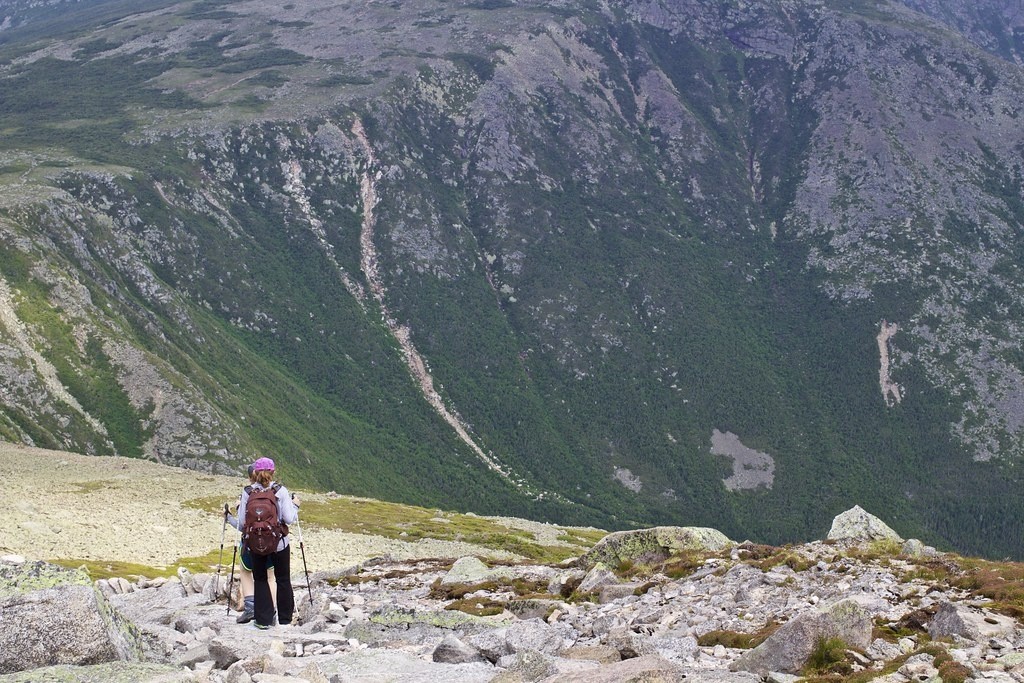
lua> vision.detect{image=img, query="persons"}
[223,463,276,611]
[238,457,301,630]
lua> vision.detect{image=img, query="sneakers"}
[236,611,255,623]
[271,615,276,626]
[253,620,269,630]
[279,618,289,624]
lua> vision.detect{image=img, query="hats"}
[255,456,274,471]
[248,464,254,474]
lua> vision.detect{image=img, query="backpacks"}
[242,483,282,556]
